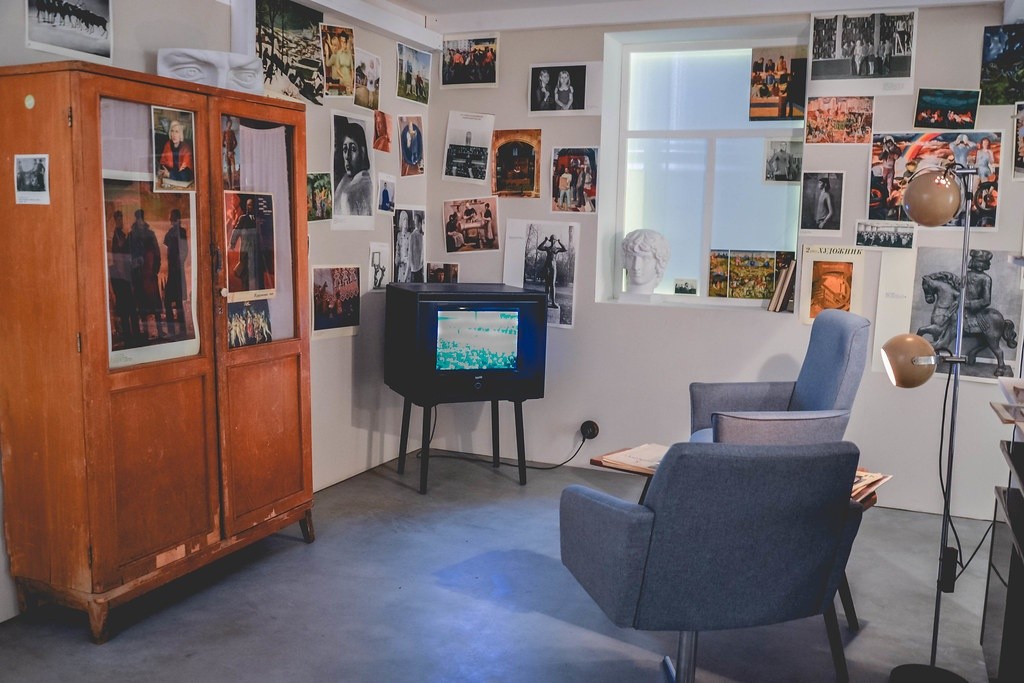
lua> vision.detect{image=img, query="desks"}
[399,397,528,494]
[591,442,877,683]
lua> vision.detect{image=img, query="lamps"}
[901,165,965,228]
[881,333,939,389]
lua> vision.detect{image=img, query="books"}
[601,443,673,474]
[850,471,894,502]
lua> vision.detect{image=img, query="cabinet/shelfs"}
[0,61,314,641]
[978,376,1024,683]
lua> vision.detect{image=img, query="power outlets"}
[582,421,599,439]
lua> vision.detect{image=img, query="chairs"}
[559,441,864,683]
[689,309,871,446]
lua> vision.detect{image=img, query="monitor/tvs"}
[384,282,547,406]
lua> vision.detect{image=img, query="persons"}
[332,289,345,323]
[437,311,517,370]
[444,47,496,86]
[857,231,913,247]
[110,207,189,348]
[405,69,425,98]
[536,235,569,308]
[816,178,834,229]
[324,30,354,97]
[395,211,425,284]
[431,268,445,284]
[228,199,274,289]
[559,158,592,210]
[752,56,794,117]
[315,184,332,219]
[373,264,386,288]
[157,120,193,190]
[537,70,574,110]
[769,141,793,182]
[364,58,376,109]
[622,228,670,294]
[382,182,391,211]
[263,47,324,97]
[375,112,391,154]
[814,15,912,76]
[228,306,271,348]
[446,202,495,252]
[16,159,45,192]
[223,117,238,191]
